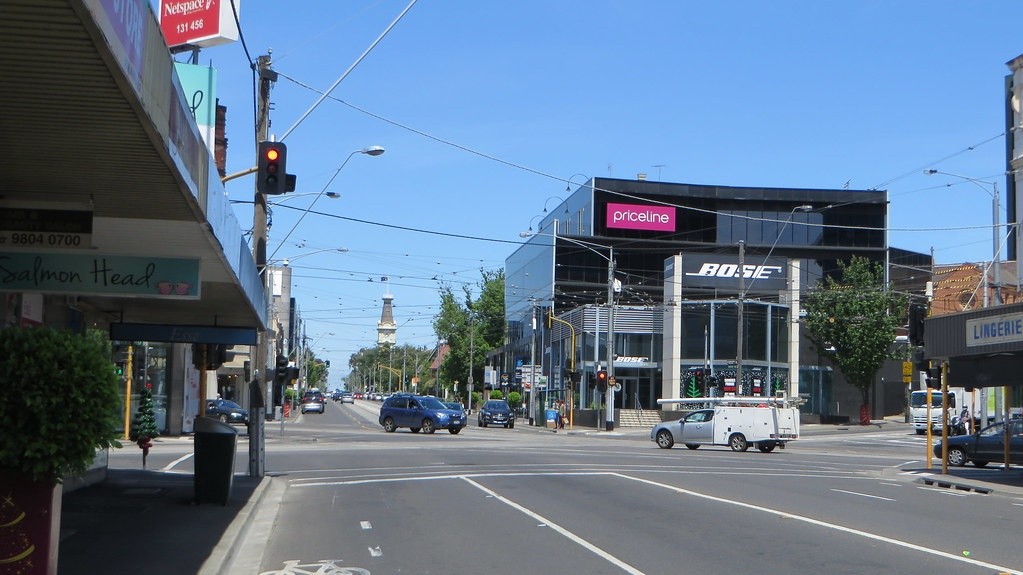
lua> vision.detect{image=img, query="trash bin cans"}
[547,410,559,430]
[275,406,283,421]
[194,415,239,505]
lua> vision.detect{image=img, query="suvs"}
[378,393,468,434]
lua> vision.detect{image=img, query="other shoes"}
[562,427,564,429]
[559,427,561,429]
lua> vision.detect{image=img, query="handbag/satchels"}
[562,417,569,424]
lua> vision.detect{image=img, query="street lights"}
[519,230,617,431]
[923,169,1000,305]
[735,204,814,394]
[266,247,349,421]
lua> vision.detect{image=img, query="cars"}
[301,391,328,414]
[331,391,354,404]
[355,392,396,402]
[478,399,515,429]
[204,398,248,424]
[933,417,1023,467]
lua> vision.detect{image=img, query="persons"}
[552,399,566,429]
[217,393,222,400]
[921,395,927,404]
[961,403,970,436]
[936,395,952,408]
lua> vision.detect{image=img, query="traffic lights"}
[596,371,607,391]
[258,141,287,194]
[277,355,290,382]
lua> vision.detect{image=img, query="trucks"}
[651,406,800,452]
[910,390,957,435]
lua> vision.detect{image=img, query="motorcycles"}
[950,417,978,436]
